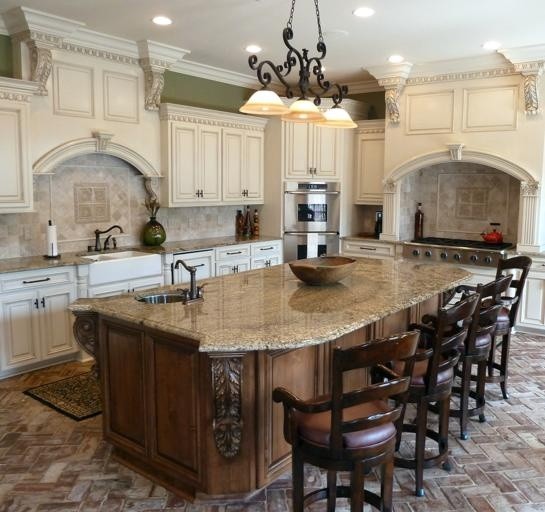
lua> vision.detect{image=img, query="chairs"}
[456,255,533,401]
[272,325,420,512]
[407,272,515,443]
[370,291,481,496]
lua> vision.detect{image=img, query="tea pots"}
[481,222,504,245]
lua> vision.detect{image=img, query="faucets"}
[94,224,124,251]
[175,259,198,300]
[177,306,203,332]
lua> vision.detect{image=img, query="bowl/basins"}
[288,256,358,286]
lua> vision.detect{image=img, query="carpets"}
[21,370,104,423]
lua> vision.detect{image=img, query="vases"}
[141,217,166,247]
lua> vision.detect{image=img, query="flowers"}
[143,192,164,219]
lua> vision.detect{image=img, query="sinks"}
[134,291,191,304]
[81,249,163,287]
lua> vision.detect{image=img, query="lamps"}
[238,1,359,129]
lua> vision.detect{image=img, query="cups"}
[88,246,95,252]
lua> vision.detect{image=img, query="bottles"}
[245,206,253,237]
[253,209,259,236]
[236,210,245,234]
[374,214,382,236]
[415,203,424,240]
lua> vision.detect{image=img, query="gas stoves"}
[402,236,512,269]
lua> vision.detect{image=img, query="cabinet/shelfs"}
[516,254,545,334]
[175,250,214,283]
[159,102,222,209]
[352,131,387,205]
[222,110,269,206]
[80,275,164,360]
[286,121,338,178]
[0,264,79,377]
[215,243,250,277]
[250,239,282,269]
[342,232,393,260]
[1,88,33,215]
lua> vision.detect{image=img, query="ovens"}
[283,180,342,262]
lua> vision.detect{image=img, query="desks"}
[81,250,475,508]
